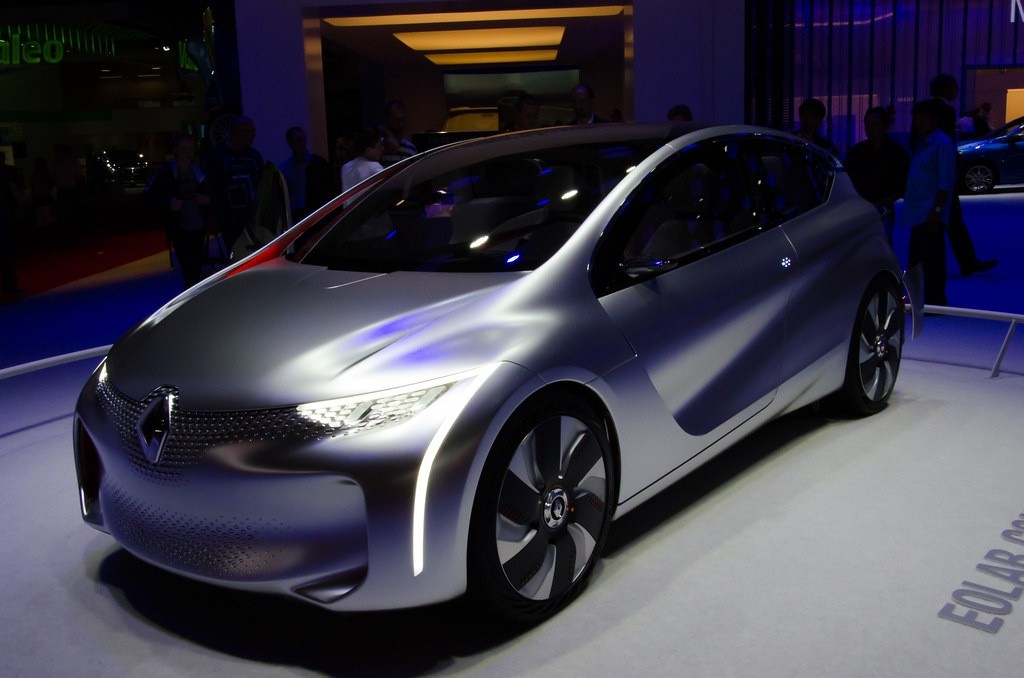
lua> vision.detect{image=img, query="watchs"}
[933,205,941,212]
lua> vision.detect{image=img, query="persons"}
[146,133,214,289]
[340,133,394,242]
[329,134,358,194]
[376,101,417,170]
[909,73,998,276]
[666,105,692,123]
[0,152,26,293]
[206,116,264,265]
[502,95,540,130]
[956,108,994,139]
[903,102,954,317]
[279,126,329,254]
[771,100,838,207]
[846,106,910,249]
[84,145,119,184]
[567,85,609,124]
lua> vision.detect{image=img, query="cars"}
[950,117,1024,193]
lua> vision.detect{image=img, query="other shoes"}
[960,259,997,277]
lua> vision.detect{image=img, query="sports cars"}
[71,118,920,634]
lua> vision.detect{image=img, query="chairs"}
[434,158,551,277]
[627,170,737,269]
